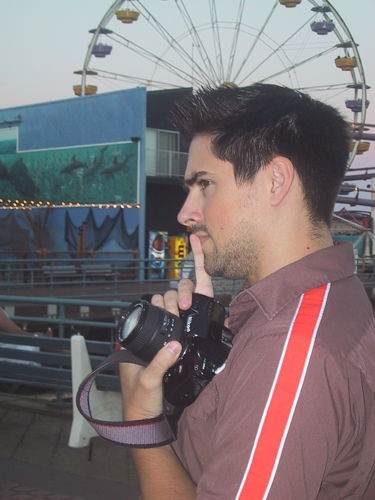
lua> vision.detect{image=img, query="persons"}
[120,84,375,500]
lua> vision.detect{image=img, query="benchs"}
[0,332,121,461]
[43,264,120,286]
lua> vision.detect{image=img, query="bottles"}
[150,232,164,275]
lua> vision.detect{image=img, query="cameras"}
[115,293,234,408]
